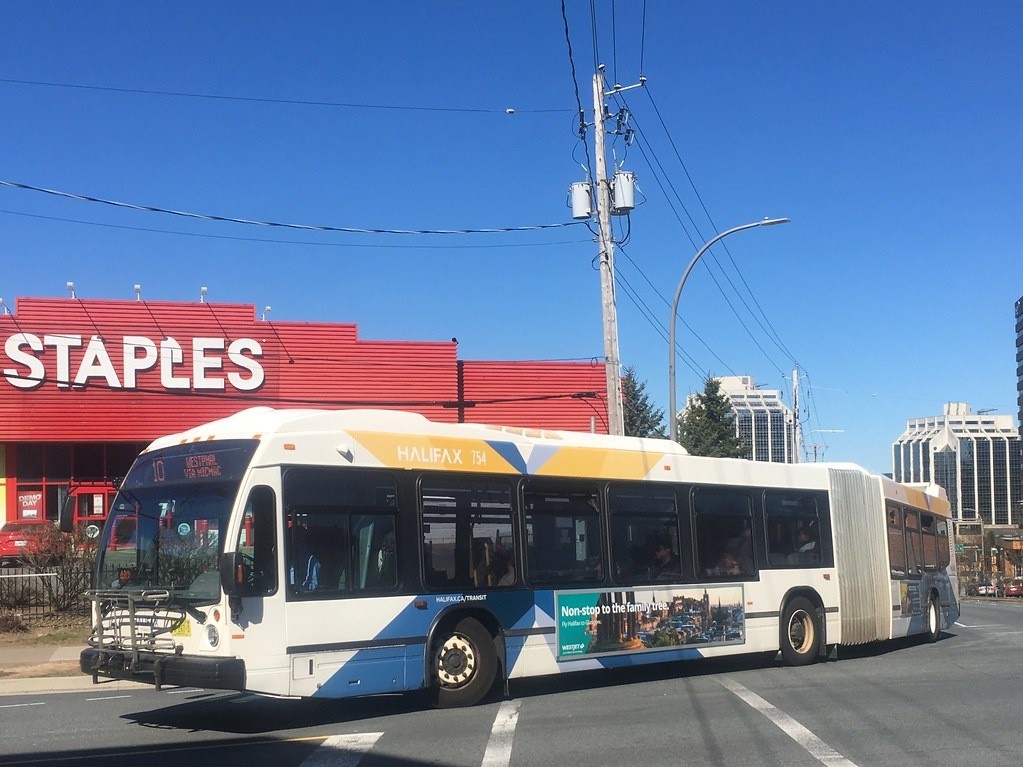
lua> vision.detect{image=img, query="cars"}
[976,582,995,596]
[0,519,66,566]
[1001,578,1023,598]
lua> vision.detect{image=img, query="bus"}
[80,407,962,708]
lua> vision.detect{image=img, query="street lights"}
[670,217,792,442]
[963,508,985,580]
[796,429,845,464]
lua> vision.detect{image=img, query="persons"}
[272,525,320,591]
[718,550,739,574]
[655,544,678,579]
[799,527,816,552]
[498,556,515,585]
